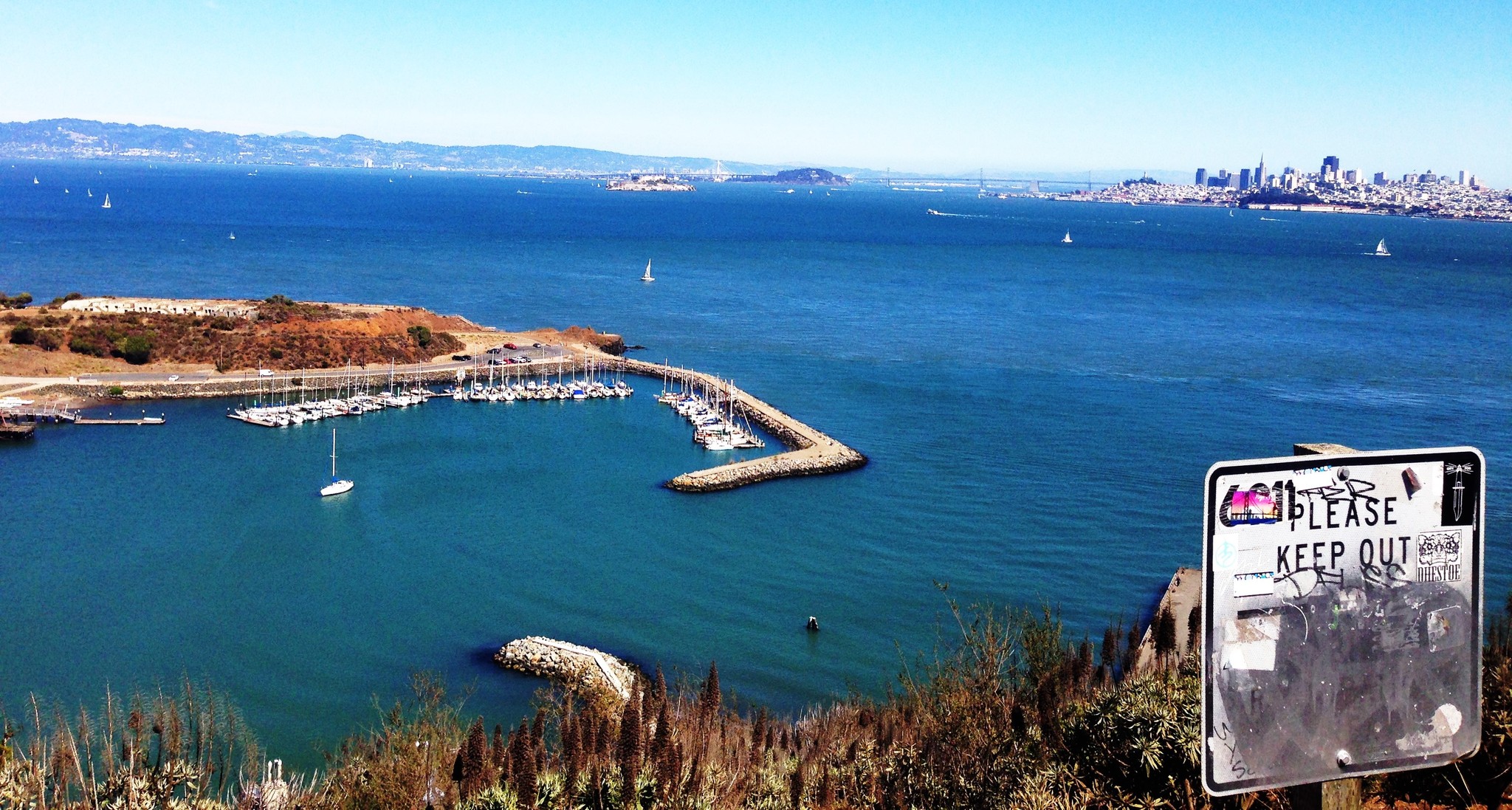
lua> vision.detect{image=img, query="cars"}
[533,343,542,347]
[488,355,532,365]
[168,375,179,381]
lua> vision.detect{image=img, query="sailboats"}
[1375,237,1391,256]
[227,232,236,239]
[640,258,655,282]
[101,193,111,209]
[597,183,601,188]
[87,188,93,197]
[409,174,412,178]
[1061,228,1073,243]
[65,187,69,193]
[1229,210,1234,217]
[591,183,594,186]
[99,171,102,174]
[389,178,393,183]
[34,175,39,184]
[827,191,831,196]
[247,169,258,176]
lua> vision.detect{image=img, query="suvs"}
[487,348,500,354]
[452,355,471,361]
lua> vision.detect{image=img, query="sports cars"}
[504,343,517,349]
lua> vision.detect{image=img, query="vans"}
[259,369,274,376]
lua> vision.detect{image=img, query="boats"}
[1260,217,1266,220]
[226,356,436,427]
[653,356,765,450]
[1140,220,1144,223]
[320,428,354,496]
[453,345,635,401]
[927,208,935,213]
